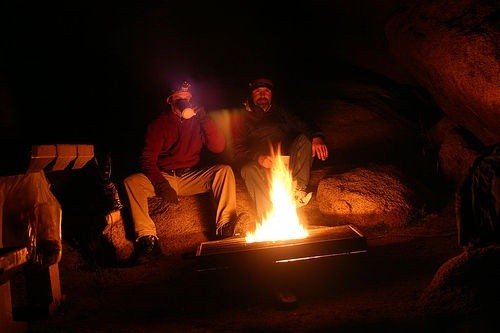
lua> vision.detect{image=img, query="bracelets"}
[313,138,319,141]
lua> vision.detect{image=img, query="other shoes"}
[218,212,250,239]
[128,236,161,265]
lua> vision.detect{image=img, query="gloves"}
[190,96,206,113]
[155,181,178,204]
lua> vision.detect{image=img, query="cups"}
[272,155,290,177]
[176,100,194,119]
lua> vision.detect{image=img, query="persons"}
[120,81,247,267]
[230,80,329,207]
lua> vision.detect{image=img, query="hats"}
[249,74,274,94]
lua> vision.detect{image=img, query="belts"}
[165,167,194,177]
